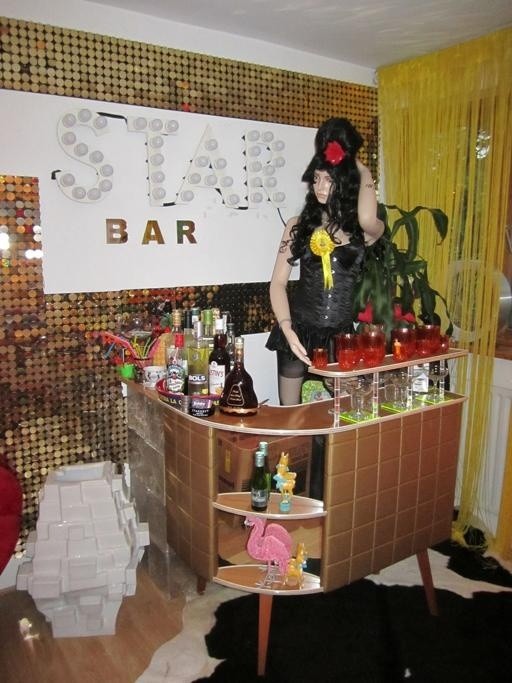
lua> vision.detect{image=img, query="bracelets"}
[279,319,292,330]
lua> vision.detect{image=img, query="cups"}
[311,345,328,372]
[143,366,167,388]
[335,325,448,367]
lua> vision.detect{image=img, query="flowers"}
[324,141,346,163]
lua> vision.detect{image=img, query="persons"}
[266,118,385,406]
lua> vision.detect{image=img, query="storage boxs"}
[216,435,313,528]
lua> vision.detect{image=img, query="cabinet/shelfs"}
[210,492,326,595]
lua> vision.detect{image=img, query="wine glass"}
[339,373,449,420]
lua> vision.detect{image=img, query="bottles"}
[166,306,259,420]
[247,441,271,512]
